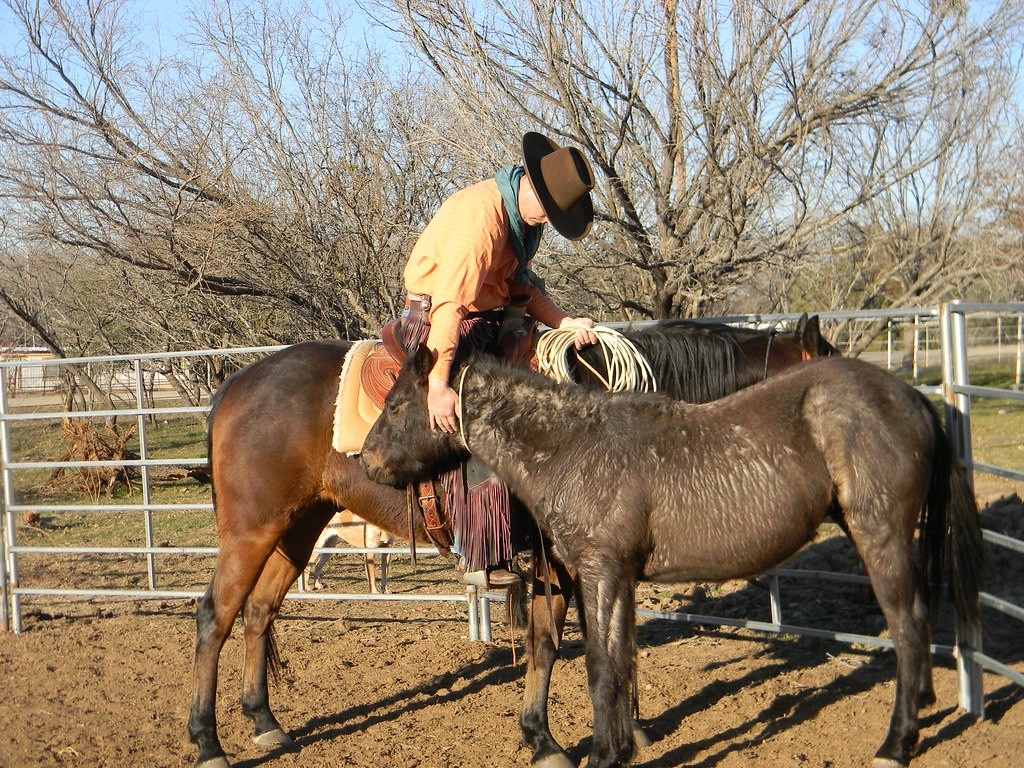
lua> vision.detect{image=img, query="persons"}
[402,132,598,587]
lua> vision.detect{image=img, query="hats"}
[522,132,595,241]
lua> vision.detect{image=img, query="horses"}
[185,315,845,767]
[360,339,987,767]
